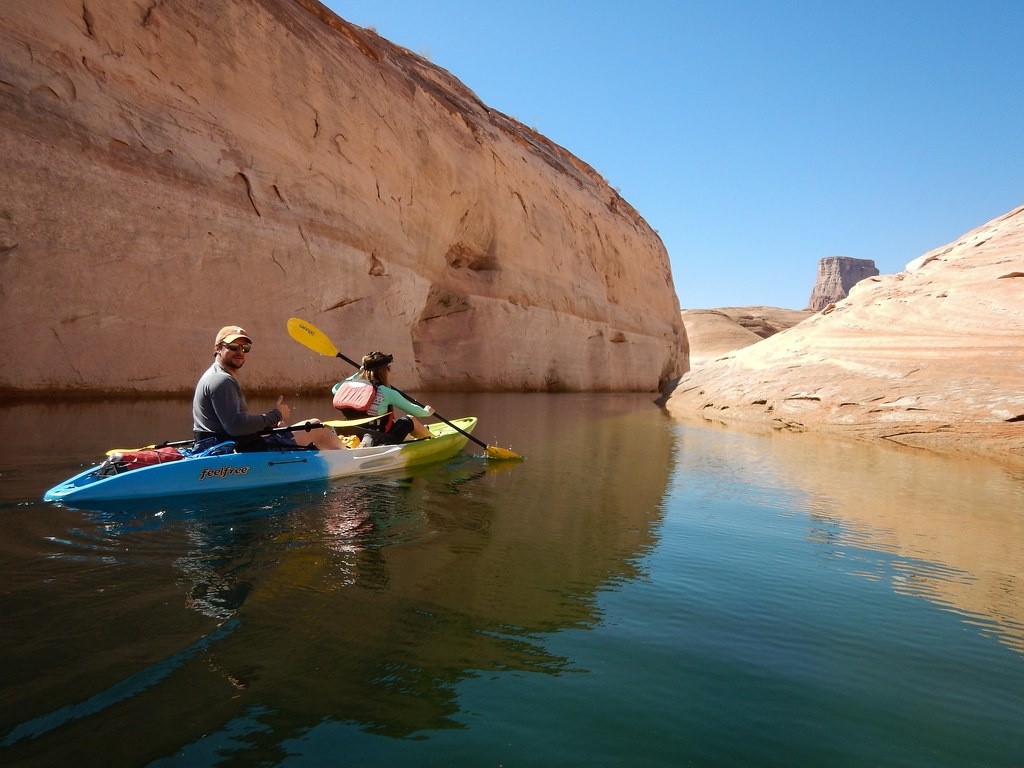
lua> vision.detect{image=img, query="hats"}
[214,325,252,347]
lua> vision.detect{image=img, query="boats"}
[47,413,476,509]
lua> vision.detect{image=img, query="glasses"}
[222,343,251,353]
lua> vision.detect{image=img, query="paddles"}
[286,316,519,459]
[104,411,393,459]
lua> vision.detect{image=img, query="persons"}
[331,351,437,445]
[192,326,347,449]
